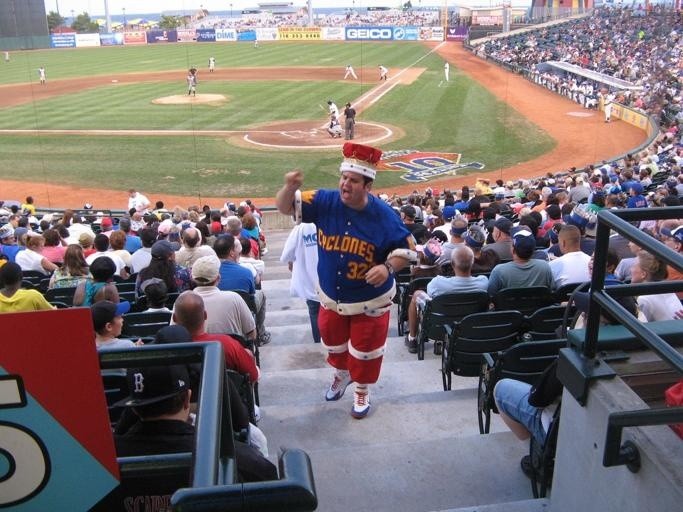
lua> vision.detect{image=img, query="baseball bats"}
[318,104,328,115]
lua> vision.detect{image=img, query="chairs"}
[20,262,261,445]
[440,310,526,391]
[527,305,578,340]
[414,291,489,360]
[491,286,551,317]
[394,259,513,335]
[476,339,567,434]
[553,282,589,304]
[528,404,561,498]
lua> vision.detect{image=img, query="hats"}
[79,217,242,409]
[339,142,384,180]
[400,177,683,257]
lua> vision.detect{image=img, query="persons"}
[344,103,356,140]
[277,142,416,418]
[209,56,215,72]
[187,72,197,96]
[445,61,449,80]
[40,67,46,84]
[477,0,682,136]
[377,175,682,446]
[255,39,258,47]
[546,134,683,188]
[327,100,342,137]
[280,223,321,343]
[0,187,277,492]
[202,9,474,27]
[345,65,357,80]
[378,64,387,79]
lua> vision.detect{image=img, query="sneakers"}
[345,382,370,418]
[325,369,352,400]
